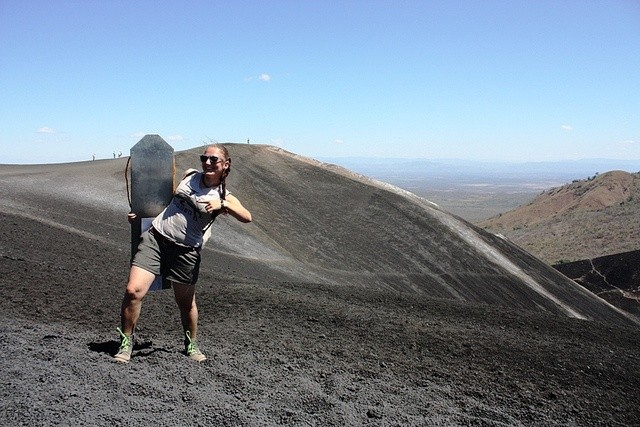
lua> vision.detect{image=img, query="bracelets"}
[220,197,225,210]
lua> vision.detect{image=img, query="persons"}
[113,145,252,364]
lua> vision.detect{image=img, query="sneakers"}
[114,327,134,363]
[184,331,207,363]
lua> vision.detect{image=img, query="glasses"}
[200,155,219,162]
